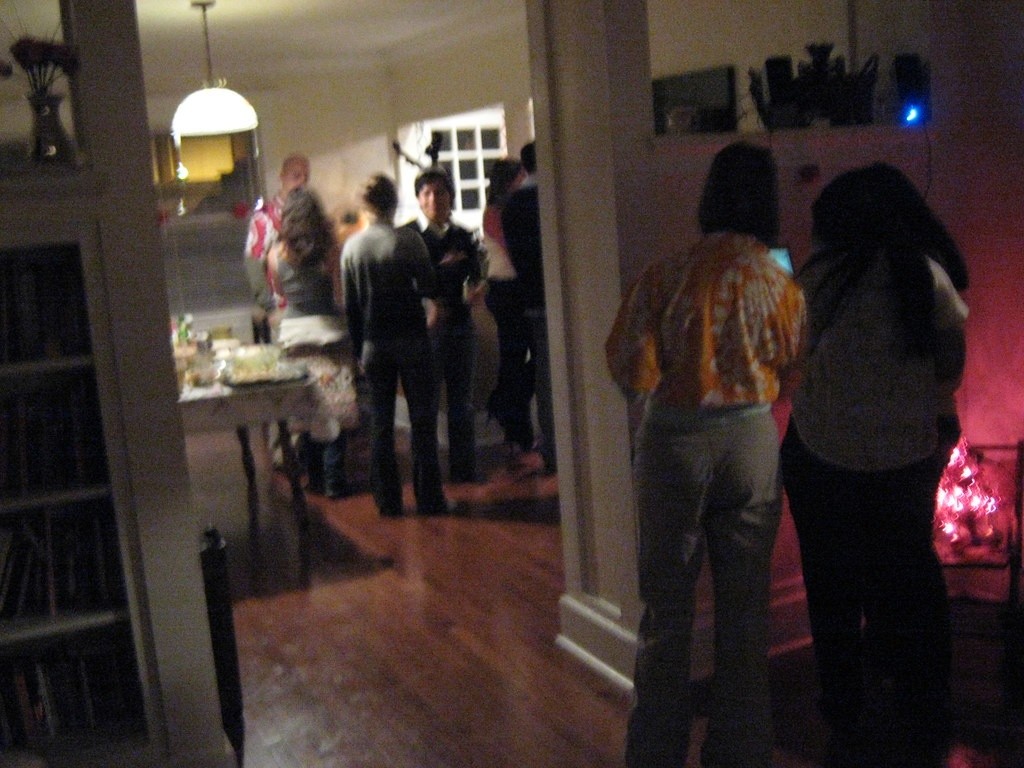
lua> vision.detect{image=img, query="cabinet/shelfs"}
[0,219,168,768]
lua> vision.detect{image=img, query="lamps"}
[170,0,258,136]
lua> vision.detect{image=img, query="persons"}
[603,143,810,767]
[245,153,352,501]
[483,140,558,477]
[785,161,970,766]
[342,165,488,516]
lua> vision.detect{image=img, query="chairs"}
[781,409,1024,747]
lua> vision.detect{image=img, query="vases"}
[26,94,70,158]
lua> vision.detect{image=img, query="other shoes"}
[540,443,557,475]
[309,480,327,492]
[380,505,404,517]
[416,501,467,514]
[327,482,361,498]
[451,466,491,484]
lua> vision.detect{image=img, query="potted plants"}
[749,42,833,128]
[827,58,878,125]
[894,50,933,123]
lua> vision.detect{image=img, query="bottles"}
[178,314,189,345]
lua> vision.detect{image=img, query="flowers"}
[0,37,81,97]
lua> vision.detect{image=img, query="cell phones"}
[768,247,795,275]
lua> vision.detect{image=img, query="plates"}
[221,367,307,388]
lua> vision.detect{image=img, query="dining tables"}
[176,339,320,580]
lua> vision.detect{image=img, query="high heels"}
[485,397,512,430]
[504,430,533,454]
